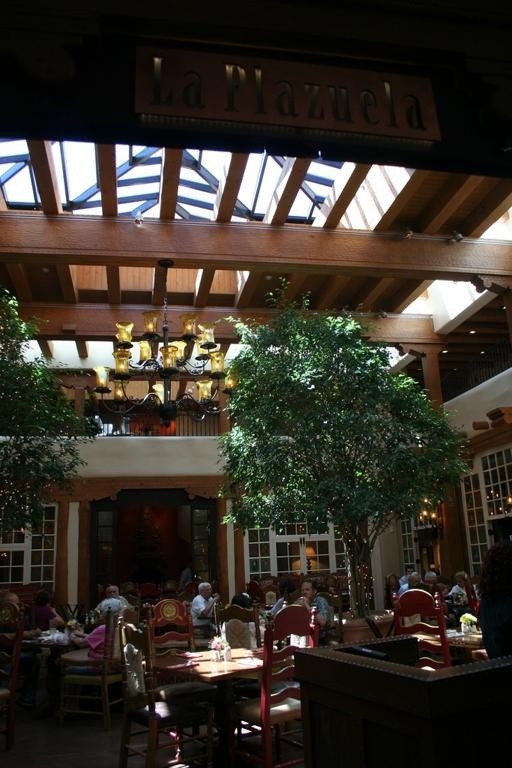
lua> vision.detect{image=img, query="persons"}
[164,579,184,600]
[231,576,296,614]
[64,613,122,676]
[97,585,134,617]
[1,593,32,633]
[300,578,332,638]
[179,564,192,589]
[191,582,220,639]
[96,582,107,603]
[384,564,479,630]
[36,591,65,631]
[477,539,510,673]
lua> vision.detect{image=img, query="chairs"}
[2,572,512,767]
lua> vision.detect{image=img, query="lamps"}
[93,260,244,428]
